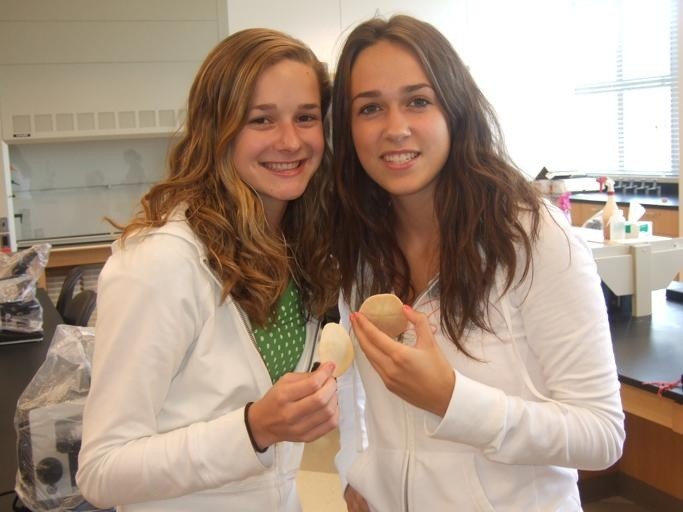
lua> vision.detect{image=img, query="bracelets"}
[243,399,268,454]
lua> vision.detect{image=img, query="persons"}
[320,14,627,512]
[72,27,340,512]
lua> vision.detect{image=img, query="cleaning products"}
[594,175,620,242]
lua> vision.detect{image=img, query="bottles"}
[556,192,573,228]
[609,209,628,241]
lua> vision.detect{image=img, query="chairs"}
[55,266,95,326]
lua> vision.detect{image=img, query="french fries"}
[359,293,409,339]
[318,321,354,379]
